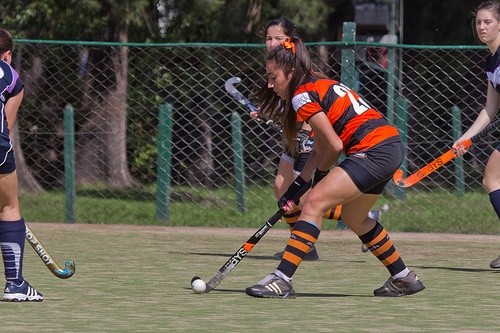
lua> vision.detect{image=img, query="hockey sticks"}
[190,204,286,294]
[392,137,479,188]
[225,77,283,136]
[25,223,76,280]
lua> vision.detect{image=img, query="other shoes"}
[490,256,500,268]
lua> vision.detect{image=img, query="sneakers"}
[362,207,381,255]
[374,270,425,297]
[2,279,43,302]
[274,243,319,261]
[246,269,297,300]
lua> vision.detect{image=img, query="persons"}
[249,17,381,259]
[450,0,500,268]
[246,35,425,300]
[0,29,43,302]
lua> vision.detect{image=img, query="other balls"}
[191,279,207,294]
